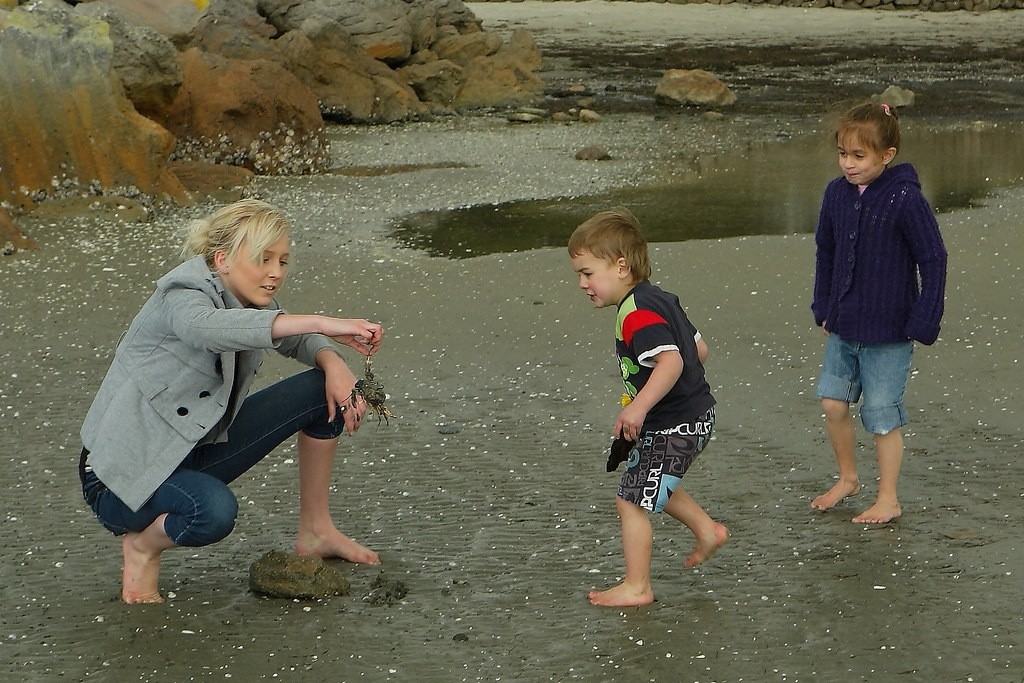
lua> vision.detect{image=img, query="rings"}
[359,400,364,404]
[339,406,348,414]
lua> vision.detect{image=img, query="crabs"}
[336,355,398,433]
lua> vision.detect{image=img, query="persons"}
[810,103,949,523]
[79,197,385,606]
[567,207,731,608]
